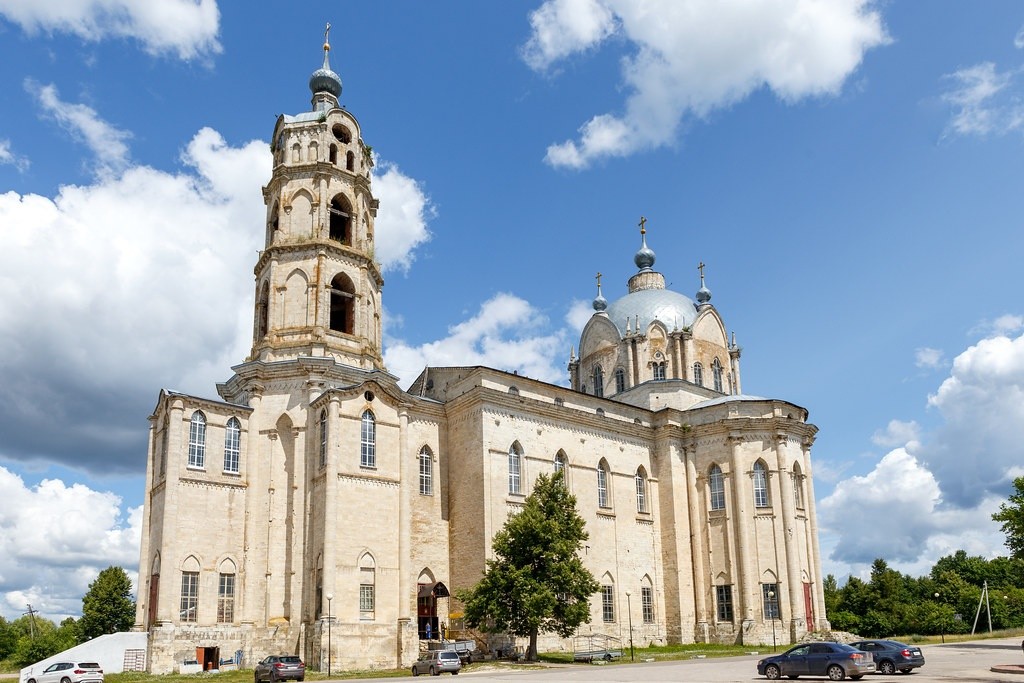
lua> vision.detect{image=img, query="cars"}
[253,653,304,683]
[757,642,877,682]
[849,640,925,675]
[412,649,462,677]
[26,661,104,683]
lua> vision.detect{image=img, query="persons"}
[425,623,432,639]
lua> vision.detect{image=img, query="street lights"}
[768,590,777,652]
[326,592,334,677]
[934,591,945,643]
[626,589,634,660]
